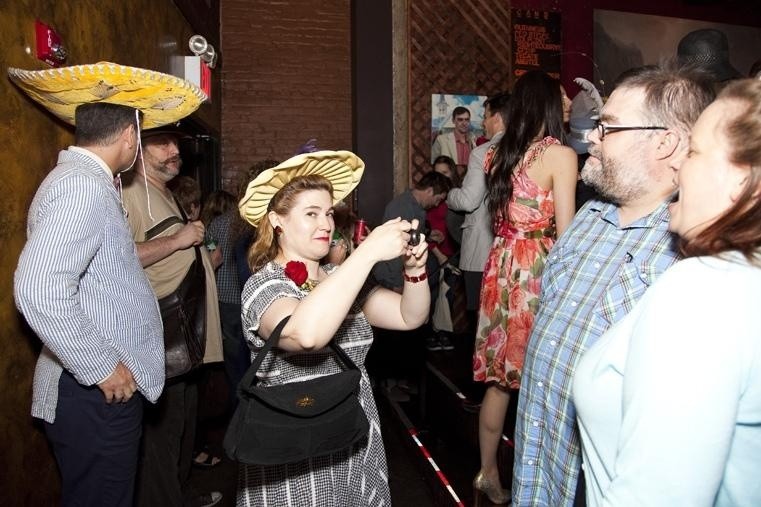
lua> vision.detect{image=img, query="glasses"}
[592,121,669,137]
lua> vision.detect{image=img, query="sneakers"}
[462,396,482,414]
[197,490,223,507]
[439,336,455,350]
[424,334,442,350]
[387,389,410,402]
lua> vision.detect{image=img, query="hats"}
[677,28,745,82]
[7,60,208,131]
[565,89,601,155]
[238,149,365,228]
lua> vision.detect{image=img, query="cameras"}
[406,229,420,245]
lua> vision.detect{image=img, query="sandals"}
[193,450,222,467]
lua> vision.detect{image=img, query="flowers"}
[285,261,308,286]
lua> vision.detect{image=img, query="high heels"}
[473,471,512,507]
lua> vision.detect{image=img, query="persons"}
[233,147,434,506]
[473,68,581,506]
[9,100,167,506]
[509,53,716,506]
[568,71,761,505]
[371,77,608,415]
[121,123,228,506]
[166,157,372,469]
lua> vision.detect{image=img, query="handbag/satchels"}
[222,314,370,466]
[158,258,207,380]
[432,281,454,332]
